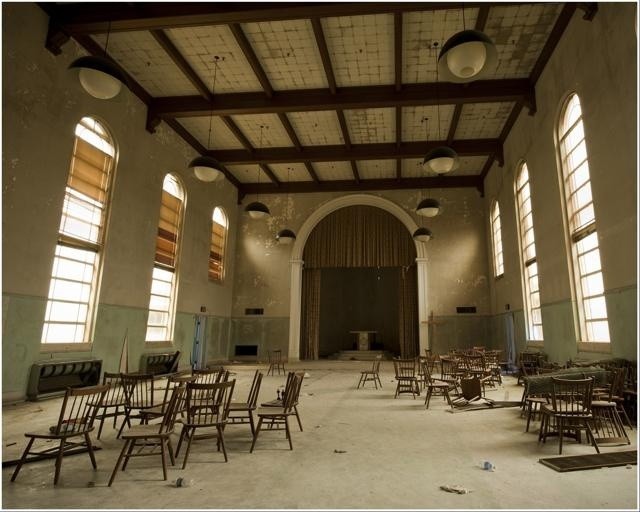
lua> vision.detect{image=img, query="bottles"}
[478,458,495,471]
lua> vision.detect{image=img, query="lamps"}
[420,41,462,178]
[245,125,273,220]
[275,168,298,245]
[414,116,445,219]
[435,4,499,88]
[412,163,435,245]
[185,55,233,184]
[68,8,132,101]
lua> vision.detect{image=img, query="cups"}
[176,477,194,487]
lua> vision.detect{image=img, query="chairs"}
[87,366,264,487]
[354,345,637,457]
[11,382,112,485]
[250,372,305,453]
[267,349,285,376]
